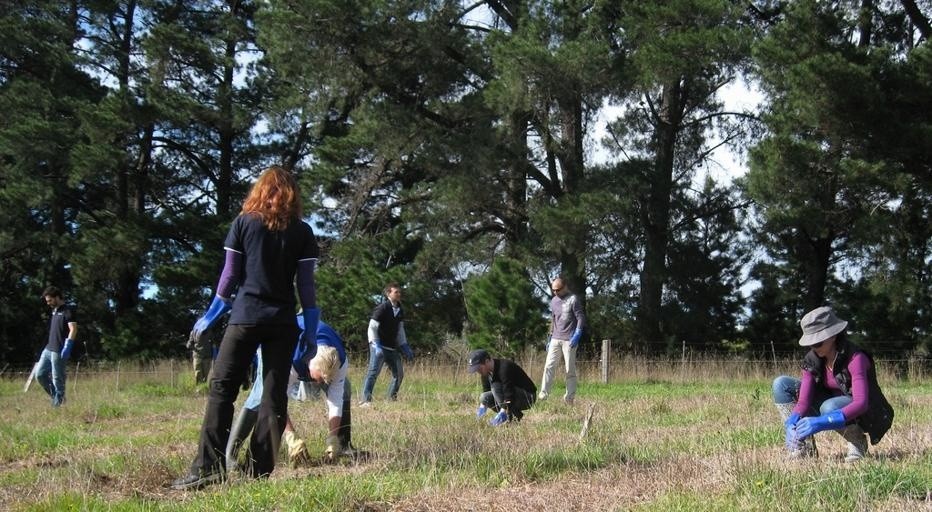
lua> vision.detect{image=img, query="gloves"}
[372,340,383,357]
[400,343,412,361]
[546,334,552,351]
[61,339,73,359]
[478,407,487,417]
[193,297,230,343]
[784,413,806,452]
[299,311,318,362]
[569,328,581,348]
[796,409,846,441]
[491,413,507,425]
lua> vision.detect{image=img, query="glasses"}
[552,288,564,292]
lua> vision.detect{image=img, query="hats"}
[798,306,848,347]
[467,349,490,373]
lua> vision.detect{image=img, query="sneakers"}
[174,471,226,490]
[784,444,818,463]
[845,449,868,462]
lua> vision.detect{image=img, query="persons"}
[186,329,211,385]
[35,285,78,407]
[772,305,894,464]
[225,309,370,475]
[170,164,320,491]
[538,278,586,403]
[467,350,537,427]
[362,282,414,404]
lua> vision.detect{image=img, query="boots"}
[337,401,369,458]
[226,407,257,472]
